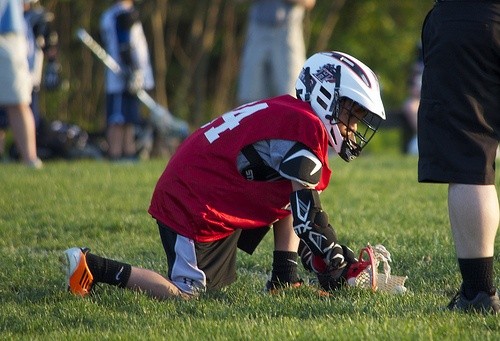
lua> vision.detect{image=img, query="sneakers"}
[442,281,500,316]
[58,247,102,296]
[264,278,332,297]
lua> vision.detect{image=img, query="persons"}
[65,50,407,301]
[100,0,155,156]
[0,0,43,168]
[417,0,500,316]
[237,0,318,104]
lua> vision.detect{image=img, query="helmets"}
[294,51,386,162]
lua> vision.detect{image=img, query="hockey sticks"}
[76,27,189,139]
[357,247,379,292]
